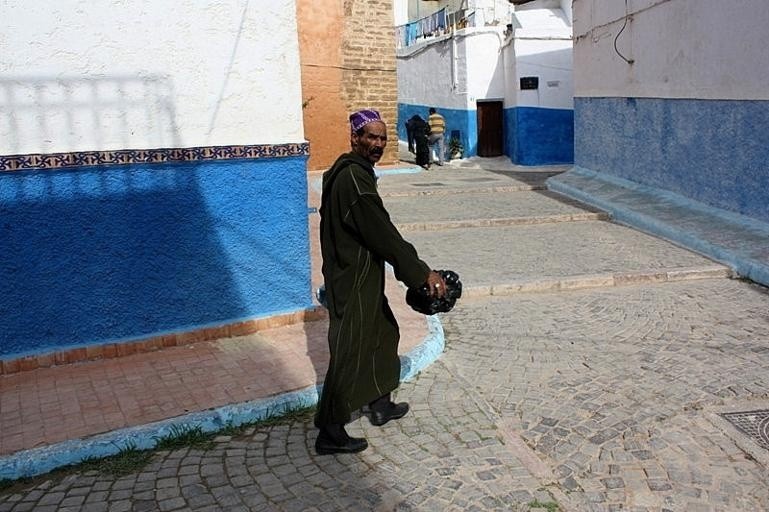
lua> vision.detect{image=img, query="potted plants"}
[448,135,464,160]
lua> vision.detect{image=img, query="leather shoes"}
[370,401,409,426]
[314,434,368,455]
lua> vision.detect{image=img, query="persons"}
[427,108,446,167]
[406,114,431,171]
[312,107,448,456]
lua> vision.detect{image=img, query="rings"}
[434,282,440,288]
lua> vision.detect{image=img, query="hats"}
[349,109,380,134]
